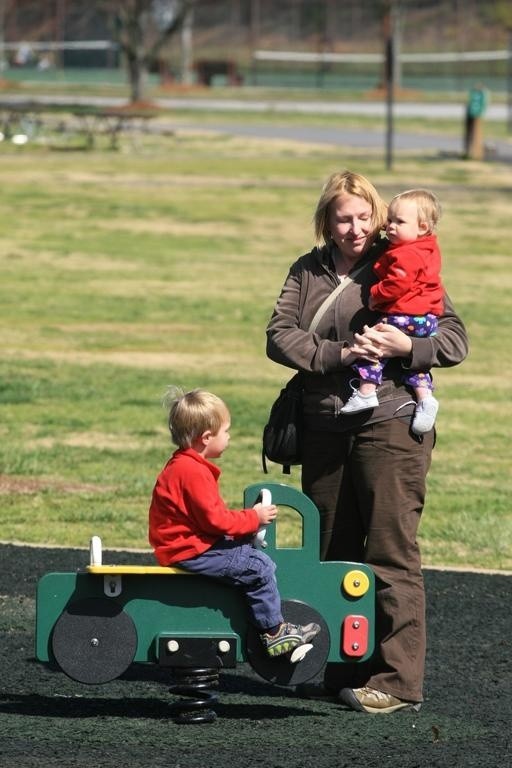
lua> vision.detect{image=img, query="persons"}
[147,388,321,658]
[265,172,472,712]
[342,187,448,432]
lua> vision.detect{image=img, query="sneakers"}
[340,392,381,416]
[338,685,419,715]
[411,397,437,436]
[261,621,322,657]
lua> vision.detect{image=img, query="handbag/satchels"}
[261,371,310,467]
